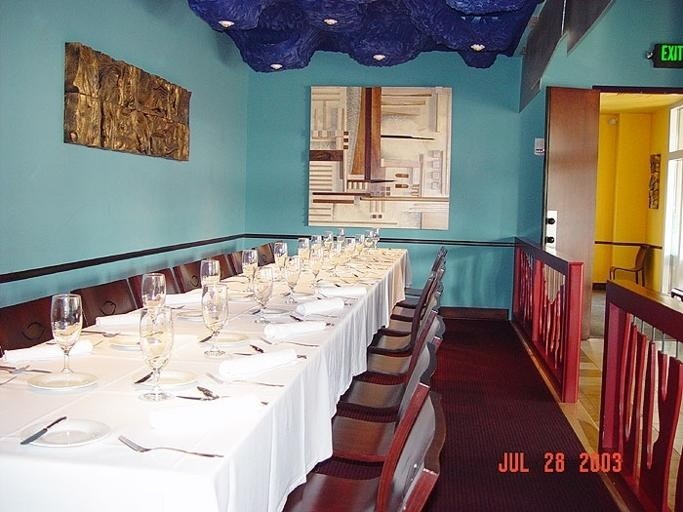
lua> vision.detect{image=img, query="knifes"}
[135,356,168,384]
[20,415,67,446]
[200,330,217,343]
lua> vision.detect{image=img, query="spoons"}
[289,314,336,326]
[198,386,269,405]
[82,330,121,337]
[251,344,307,358]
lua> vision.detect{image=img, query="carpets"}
[312,318,622,512]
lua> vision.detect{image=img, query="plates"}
[251,307,290,316]
[25,418,112,446]
[176,310,204,321]
[208,330,249,343]
[28,368,98,390]
[0,357,28,384]
[107,334,140,347]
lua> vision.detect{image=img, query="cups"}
[201,259,220,288]
[142,273,167,310]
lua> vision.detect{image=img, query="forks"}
[119,435,223,458]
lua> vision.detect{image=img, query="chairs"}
[608,243,649,287]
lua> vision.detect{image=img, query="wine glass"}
[283,256,301,305]
[250,265,273,324]
[242,226,379,287]
[201,284,230,356]
[52,293,83,373]
[139,305,174,401]
